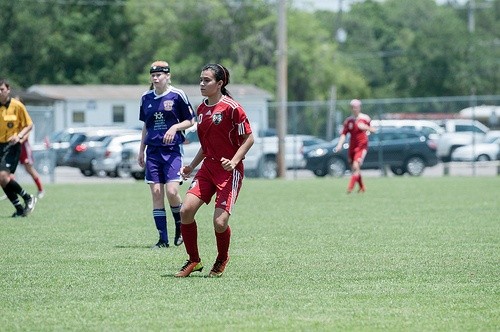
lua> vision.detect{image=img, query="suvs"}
[299,129,440,178]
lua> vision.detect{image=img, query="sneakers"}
[13,213,20,217]
[174,259,203,277]
[22,195,37,217]
[154,241,168,249]
[174,225,184,246]
[209,255,229,278]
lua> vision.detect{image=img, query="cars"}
[30,117,500,181]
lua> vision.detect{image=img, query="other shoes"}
[357,188,364,192]
[37,191,44,198]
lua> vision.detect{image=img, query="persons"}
[173,63,254,277]
[335,98,375,194]
[0,80,45,217]
[138,61,197,249]
